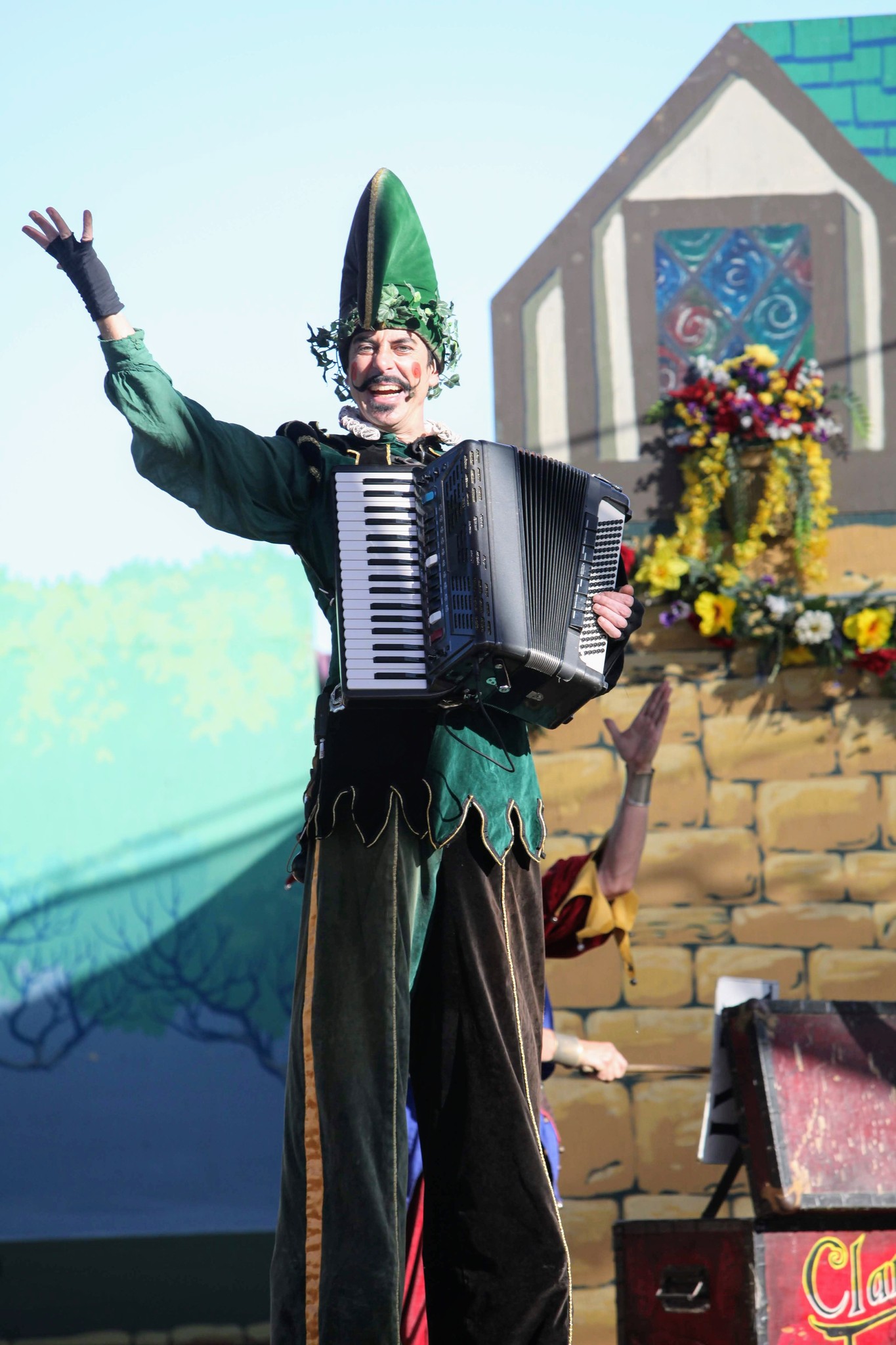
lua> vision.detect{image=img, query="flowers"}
[630,342,896,691]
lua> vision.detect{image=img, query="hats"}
[337,168,444,375]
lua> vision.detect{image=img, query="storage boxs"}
[610,997,896,1345]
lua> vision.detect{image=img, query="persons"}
[21,168,645,1345]
[403,680,672,1345]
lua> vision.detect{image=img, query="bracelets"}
[551,1032,581,1070]
[625,769,655,806]
